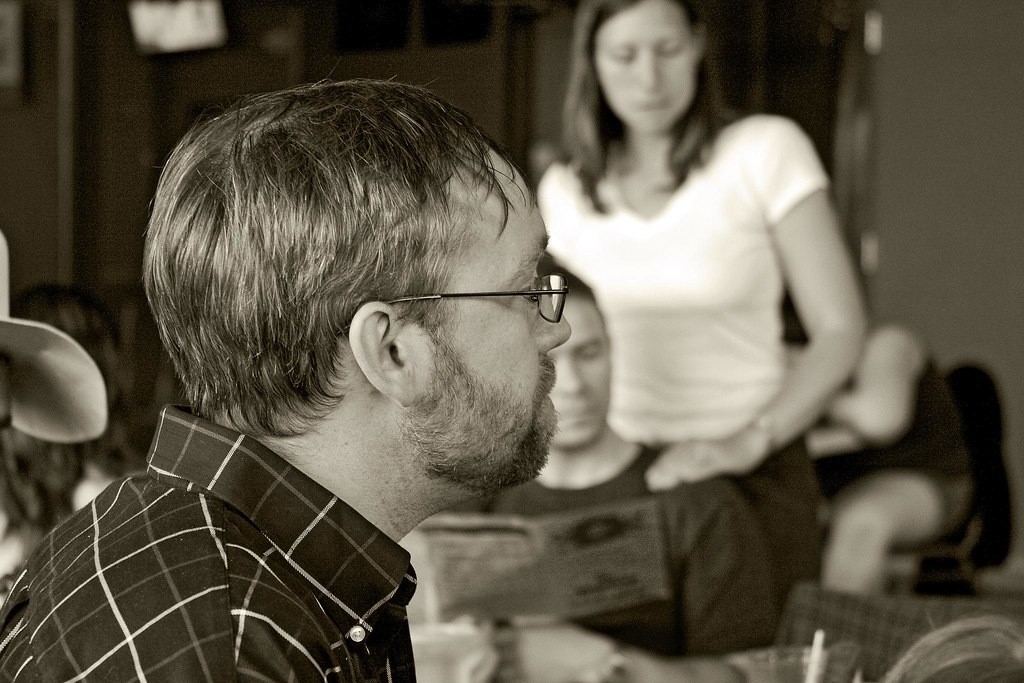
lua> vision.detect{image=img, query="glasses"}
[333,270,568,338]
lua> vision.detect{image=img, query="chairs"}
[889,363,1014,599]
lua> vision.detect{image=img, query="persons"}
[0,80,574,683]
[434,0,975,683]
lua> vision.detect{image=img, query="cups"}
[731,644,855,683]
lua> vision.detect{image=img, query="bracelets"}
[609,645,628,683]
[751,419,777,453]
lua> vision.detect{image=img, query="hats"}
[0,233,110,444]
